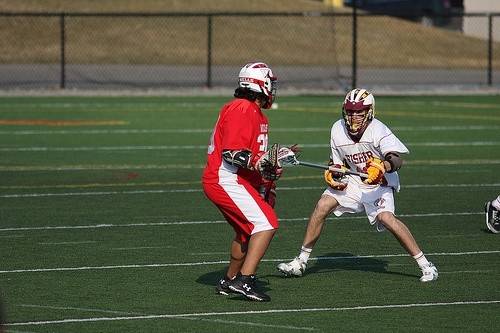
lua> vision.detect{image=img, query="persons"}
[202,62,283,303]
[278,89,439,283]
[485,195,500,233]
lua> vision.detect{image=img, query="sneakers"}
[216,274,243,295]
[484,201,500,234]
[227,271,271,301]
[419,261,438,283]
[277,257,308,278]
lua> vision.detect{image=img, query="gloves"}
[324,164,351,191]
[252,151,283,180]
[360,156,386,185]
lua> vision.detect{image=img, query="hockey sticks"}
[278,147,369,177]
[262,140,278,202]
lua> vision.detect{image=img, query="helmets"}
[237,62,278,109]
[342,88,376,139]
